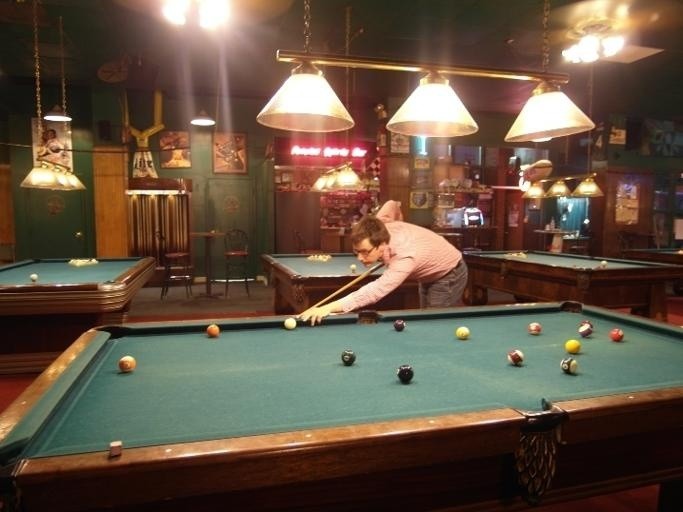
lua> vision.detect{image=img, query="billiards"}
[560,357,577,374]
[507,349,523,366]
[307,254,332,264]
[30,273,38,282]
[397,364,414,382]
[565,339,580,354]
[350,264,356,271]
[456,326,469,339]
[207,323,220,337]
[119,355,136,372]
[394,319,406,331]
[602,260,607,267]
[69,258,97,266]
[284,317,296,329]
[610,328,623,342]
[518,469,542,494]
[342,349,355,365]
[577,320,593,337]
[527,322,541,335]
[504,252,527,258]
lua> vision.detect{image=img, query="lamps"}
[189,107,215,126]
[562,18,626,64]
[42,104,72,122]
[310,159,364,191]
[521,171,604,200]
[502,80,596,143]
[19,156,87,190]
[385,70,479,137]
[256,63,356,133]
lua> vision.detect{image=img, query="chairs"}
[152,231,193,299]
[224,230,250,300]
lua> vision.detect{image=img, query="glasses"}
[351,245,377,258]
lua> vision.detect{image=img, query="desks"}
[620,248,683,264]
[461,249,683,322]
[190,232,227,301]
[1,299,683,509]
[0,256,156,376]
[260,250,421,316]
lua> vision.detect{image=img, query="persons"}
[37,129,65,161]
[301,200,469,326]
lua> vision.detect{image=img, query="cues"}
[296,261,385,318]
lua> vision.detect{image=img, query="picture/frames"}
[158,129,191,168]
[388,129,410,155]
[212,130,249,175]
[409,155,431,209]
